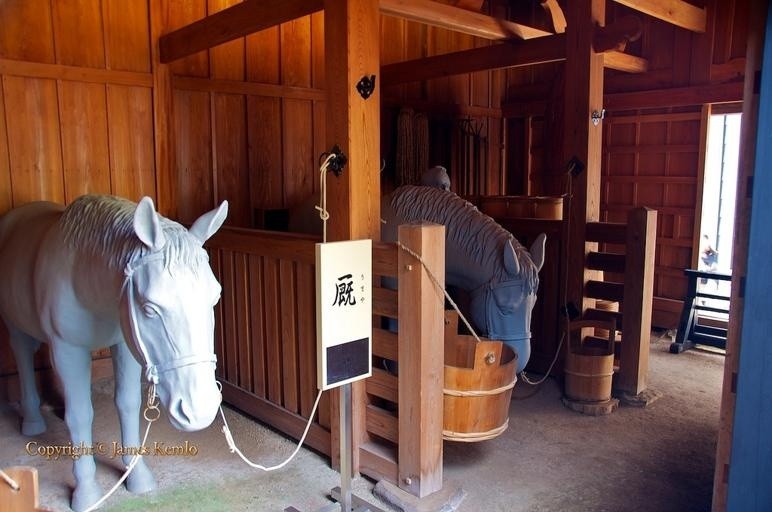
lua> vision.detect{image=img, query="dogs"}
[302,184,550,449]
[0,191,231,512]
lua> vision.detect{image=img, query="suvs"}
[395,309,519,444]
[0,467,53,512]
[564,317,616,404]
